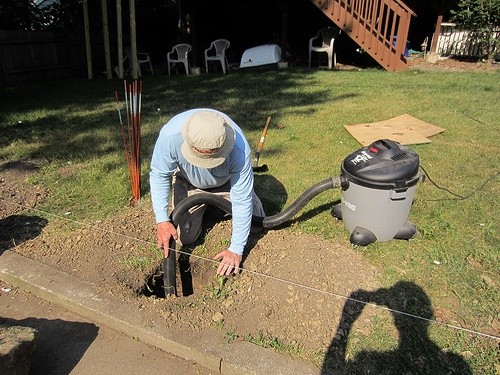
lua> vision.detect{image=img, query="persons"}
[149,108,267,277]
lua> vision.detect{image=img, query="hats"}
[181,111,236,169]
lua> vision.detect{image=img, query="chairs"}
[167,44,192,77]
[204,39,230,75]
[137,53,153,77]
[309,35,337,70]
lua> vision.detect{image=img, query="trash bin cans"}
[392,36,410,57]
